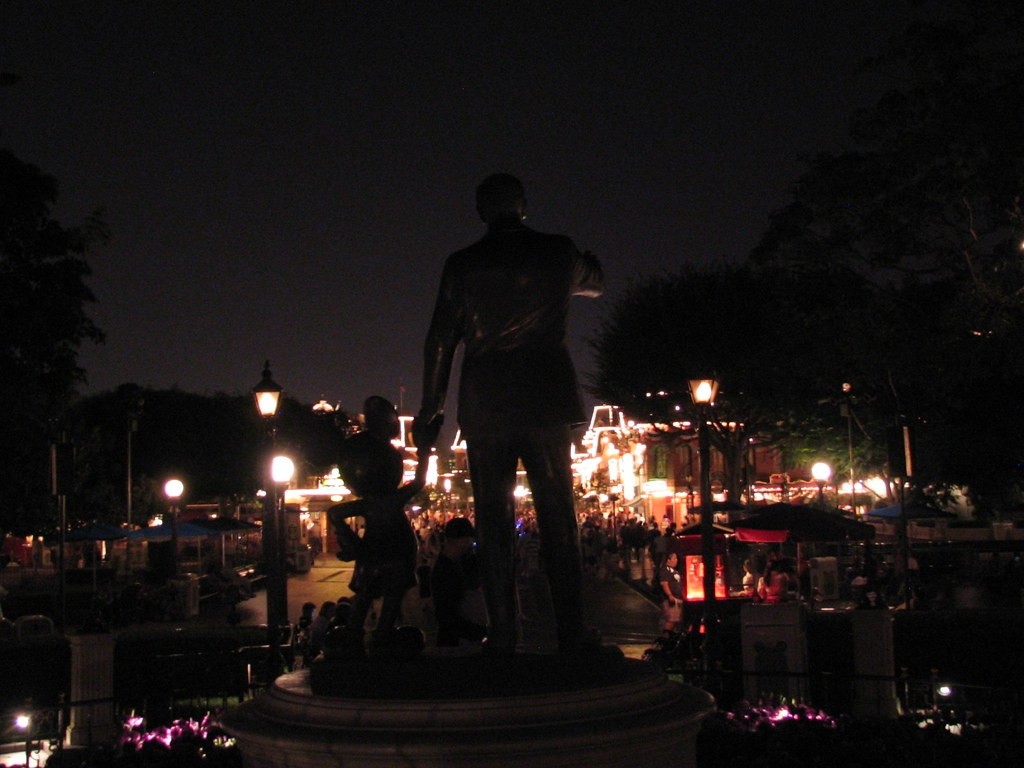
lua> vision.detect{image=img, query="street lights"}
[250,367,281,652]
[18,714,30,768]
[811,462,830,510]
[270,454,294,626]
[164,479,183,613]
[687,368,721,691]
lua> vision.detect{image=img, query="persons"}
[31,535,102,572]
[400,503,789,647]
[842,548,1024,609]
[291,596,352,671]
[410,173,607,657]
[324,419,432,656]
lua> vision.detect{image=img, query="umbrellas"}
[46,516,262,594]
[726,503,876,580]
